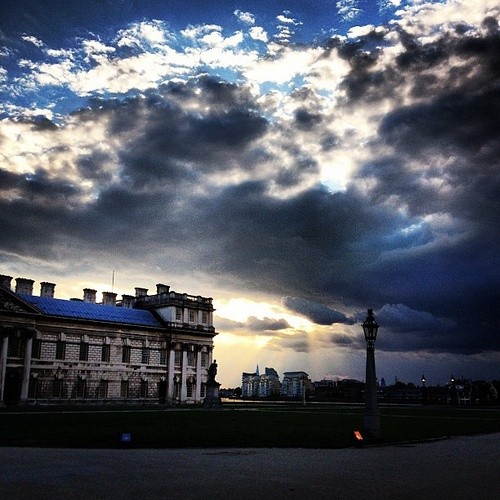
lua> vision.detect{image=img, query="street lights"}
[360,308,381,447]
[421,375,426,388]
